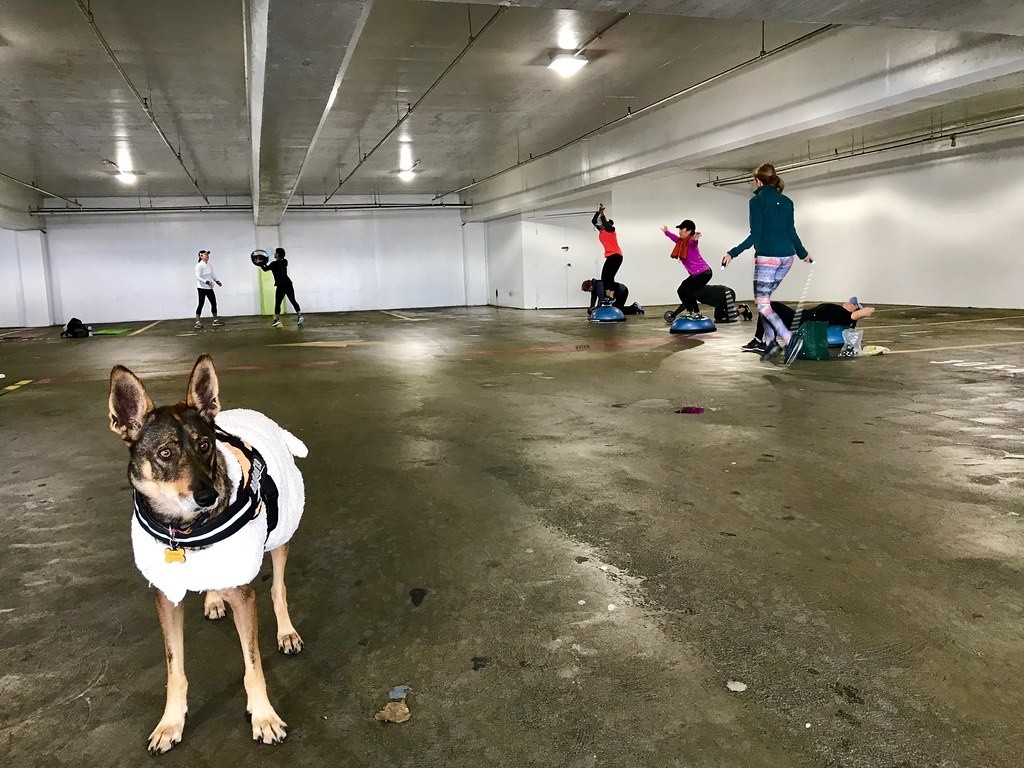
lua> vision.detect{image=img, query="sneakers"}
[600,295,617,307]
[679,311,702,320]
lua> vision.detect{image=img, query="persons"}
[582,279,644,315]
[722,164,814,364]
[193,250,225,329]
[660,220,713,320]
[668,285,753,322]
[591,207,623,307]
[741,296,875,352]
[256,248,304,326]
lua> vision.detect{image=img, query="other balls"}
[250,249,269,266]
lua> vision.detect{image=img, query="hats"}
[198,250,210,257]
[849,297,863,309]
[676,220,695,230]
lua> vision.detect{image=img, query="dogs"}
[109,352,309,756]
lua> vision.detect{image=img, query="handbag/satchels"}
[837,328,864,357]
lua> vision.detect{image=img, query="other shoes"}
[738,303,752,320]
[742,337,761,351]
[194,322,202,328]
[783,334,803,363]
[762,341,782,360]
[757,341,766,351]
[272,319,281,326]
[634,302,644,314]
[212,319,225,326]
[298,315,304,325]
